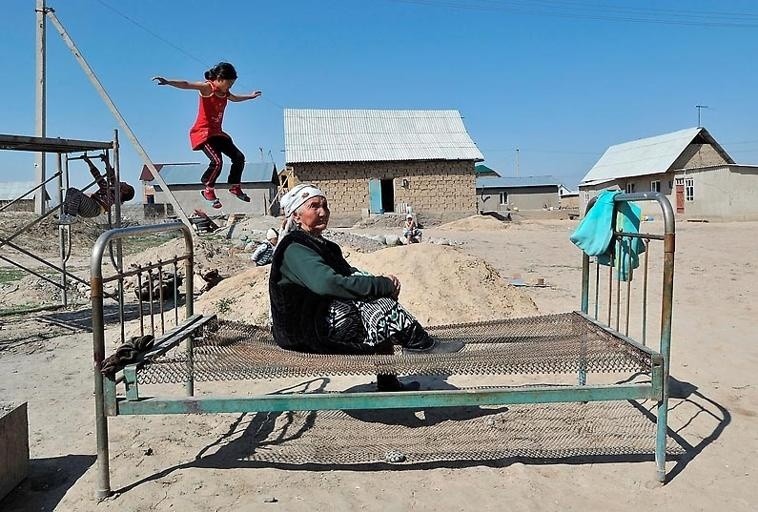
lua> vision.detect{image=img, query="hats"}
[267,227,278,240]
[279,184,326,218]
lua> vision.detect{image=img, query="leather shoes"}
[402,338,466,355]
[377,381,421,391]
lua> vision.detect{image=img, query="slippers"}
[200,190,222,210]
[228,188,252,203]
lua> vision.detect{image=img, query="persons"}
[401,214,425,245]
[271,183,466,395]
[153,62,262,210]
[252,228,278,267]
